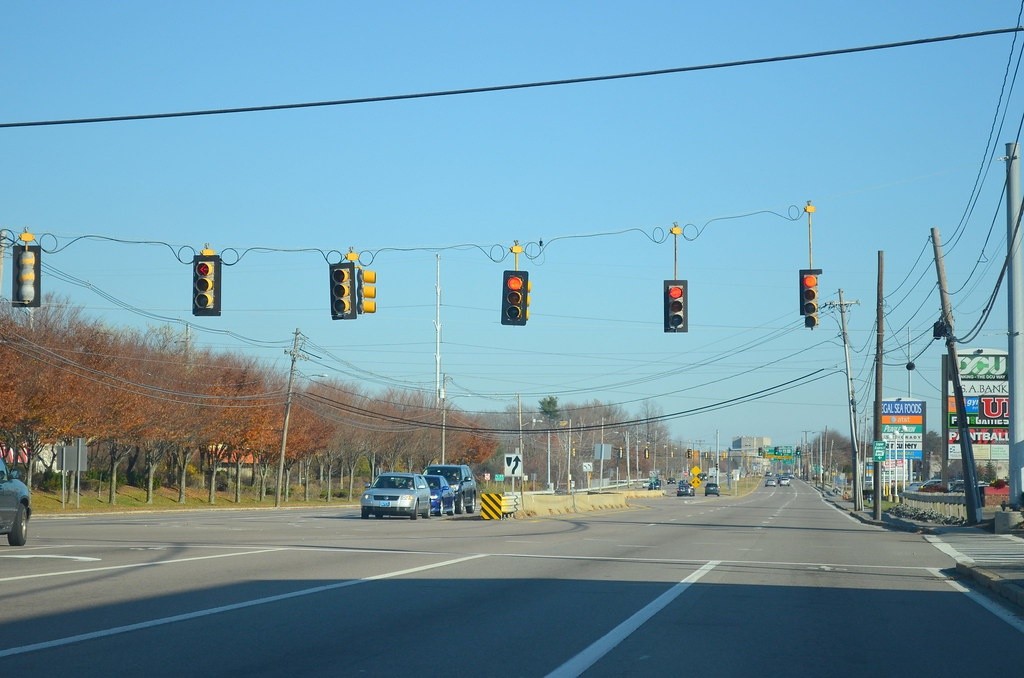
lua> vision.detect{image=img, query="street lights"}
[442,394,471,465]
[600,432,641,492]
[275,374,328,508]
[519,420,543,455]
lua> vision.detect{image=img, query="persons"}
[406,478,412,486]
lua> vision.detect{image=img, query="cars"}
[765,472,794,487]
[643,472,720,497]
[0,456,32,547]
[908,480,989,493]
[360,465,477,520]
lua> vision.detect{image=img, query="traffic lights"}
[716,463,718,471]
[501,270,532,326]
[687,449,692,458]
[330,263,357,320]
[664,280,688,332]
[759,447,762,456]
[799,269,822,328]
[193,255,221,316]
[775,447,778,455]
[796,447,800,454]
[357,269,376,314]
